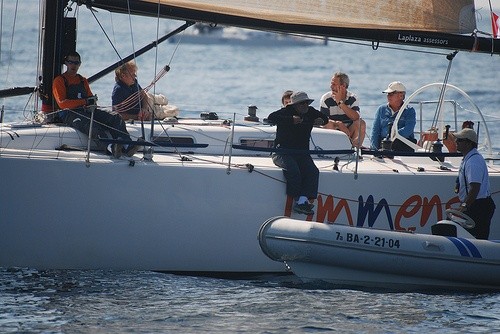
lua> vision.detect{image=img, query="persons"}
[111,62,178,120]
[371,81,416,152]
[462,121,472,128]
[52,51,143,159]
[268,91,329,215]
[282,90,294,107]
[452,128,496,240]
[320,72,366,148]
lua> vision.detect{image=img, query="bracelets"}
[459,203,469,210]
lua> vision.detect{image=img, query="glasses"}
[68,60,79,65]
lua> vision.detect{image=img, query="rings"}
[146,116,148,118]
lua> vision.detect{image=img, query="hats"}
[382,81,407,93]
[452,128,477,143]
[287,91,314,106]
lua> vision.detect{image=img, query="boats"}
[164,23,323,46]
[256,209,500,295]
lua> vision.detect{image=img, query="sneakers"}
[295,201,314,214]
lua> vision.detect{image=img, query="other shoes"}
[126,138,142,155]
[111,137,122,158]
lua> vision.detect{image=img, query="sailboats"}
[0,0,500,279]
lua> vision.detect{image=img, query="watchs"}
[338,99,343,106]
